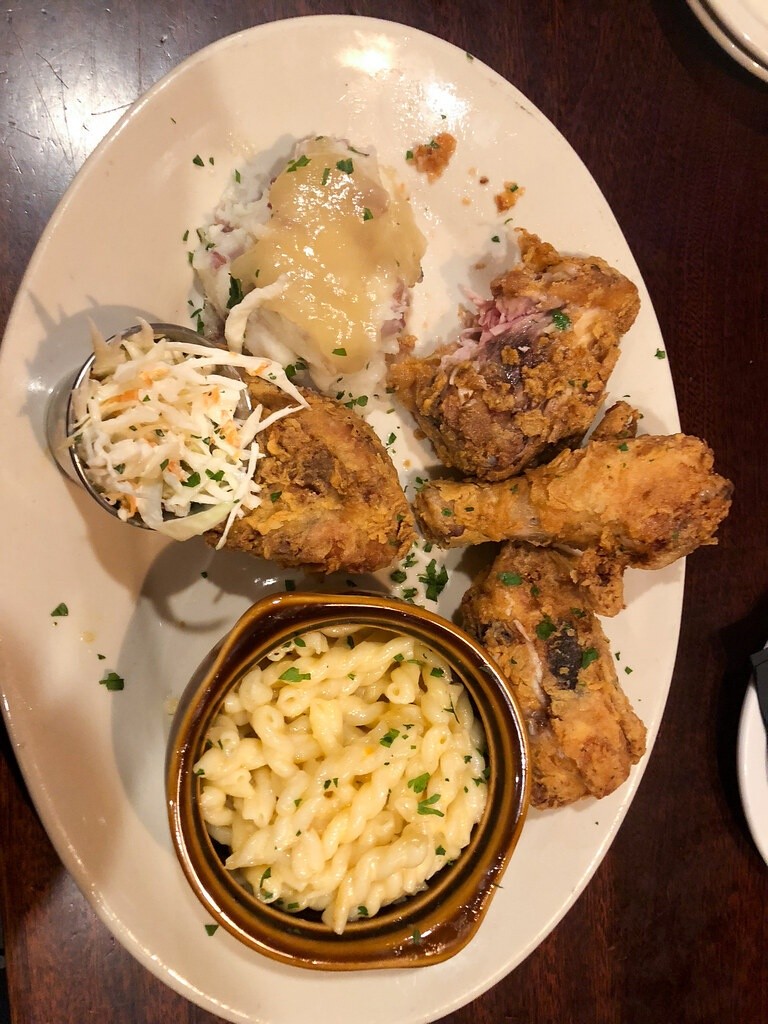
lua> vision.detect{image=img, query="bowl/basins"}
[65,324,254,530]
[165,594,531,973]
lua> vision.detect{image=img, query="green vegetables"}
[49,136,610,820]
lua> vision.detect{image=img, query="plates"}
[0,14,687,1024]
[738,638,768,869]
[686,0,768,84]
